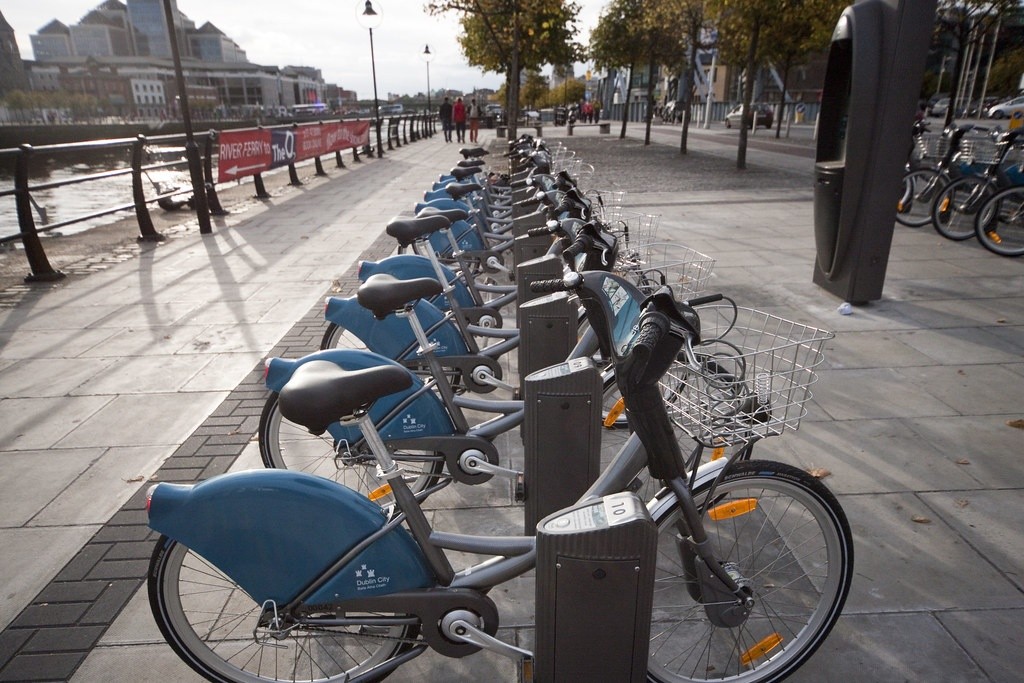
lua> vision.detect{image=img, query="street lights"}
[356,0,384,157]
[419,43,436,138]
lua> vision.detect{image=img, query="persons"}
[440,97,482,143]
[569,98,602,124]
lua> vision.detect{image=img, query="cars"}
[986,95,1024,120]
[931,97,977,117]
[725,102,773,129]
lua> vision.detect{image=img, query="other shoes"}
[449,139,452,143]
[474,141,478,143]
[470,139,473,143]
[458,138,460,143]
[445,138,448,143]
[462,138,465,144]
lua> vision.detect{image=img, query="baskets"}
[564,162,595,197]
[659,305,835,447]
[1000,148,1024,185]
[616,243,717,308]
[596,210,661,268]
[546,141,583,177]
[583,188,628,232]
[917,134,951,160]
[958,138,1009,165]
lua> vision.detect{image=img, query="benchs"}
[567,123,610,136]
[497,126,542,138]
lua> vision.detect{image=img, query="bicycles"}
[895,112,1024,257]
[147,133,856,683]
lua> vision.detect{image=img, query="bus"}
[378,104,403,115]
